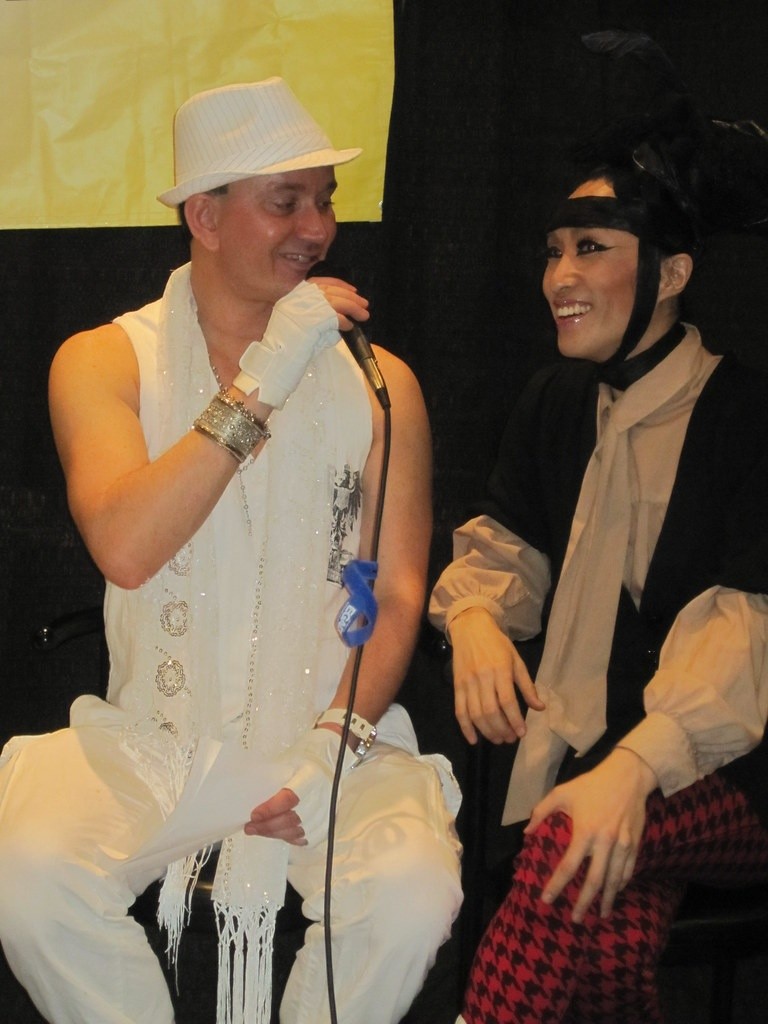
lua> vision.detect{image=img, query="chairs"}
[30,610,767,1024]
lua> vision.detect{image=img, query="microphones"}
[307,260,391,409]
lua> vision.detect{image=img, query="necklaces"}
[211,361,258,536]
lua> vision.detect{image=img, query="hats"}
[545,116,766,265]
[159,78,364,206]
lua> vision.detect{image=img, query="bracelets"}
[193,394,270,465]
[312,708,378,769]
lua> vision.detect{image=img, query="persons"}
[0,84,467,1024]
[427,168,768,1018]
